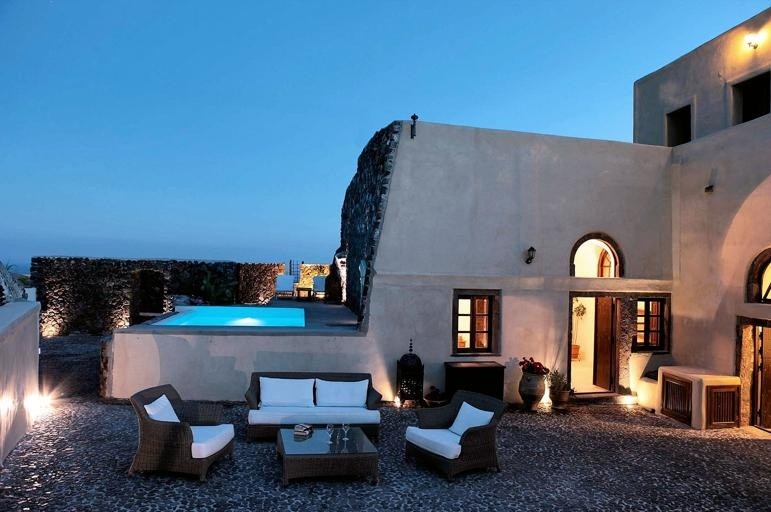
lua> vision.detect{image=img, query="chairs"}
[404,388,508,487]
[126,383,239,483]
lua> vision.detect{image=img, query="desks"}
[444,360,507,404]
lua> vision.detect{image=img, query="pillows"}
[141,395,181,424]
[448,401,495,437]
[259,377,371,409]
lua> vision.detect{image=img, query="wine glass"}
[327,424,334,443]
[341,422,350,440]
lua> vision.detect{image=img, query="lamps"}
[526,246,536,264]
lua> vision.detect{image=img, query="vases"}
[549,387,570,411]
[422,396,447,407]
[518,374,545,411]
[571,345,580,359]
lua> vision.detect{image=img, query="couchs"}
[244,370,384,442]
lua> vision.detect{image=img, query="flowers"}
[549,370,570,390]
[426,385,444,400]
[519,356,549,374]
